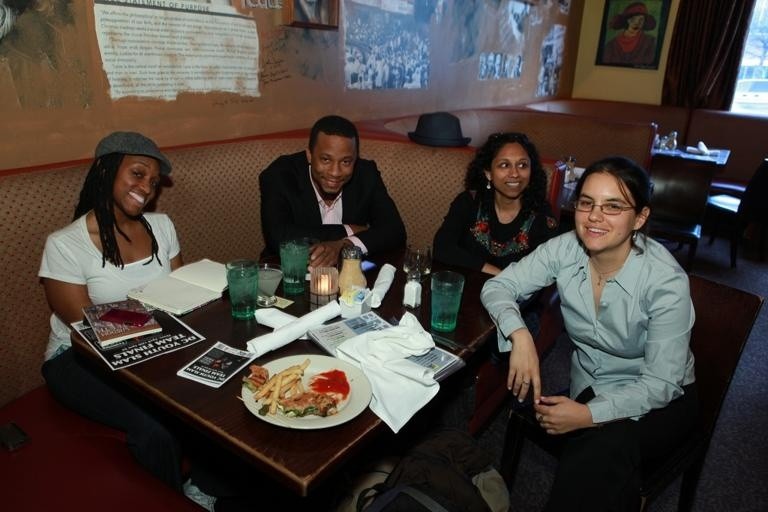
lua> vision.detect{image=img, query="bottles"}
[667,132,677,150]
[337,246,368,301]
[402,270,422,312]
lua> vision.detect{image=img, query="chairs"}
[704,157,768,268]
[503,272,763,511]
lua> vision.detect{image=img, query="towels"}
[335,312,440,434]
[369,263,397,308]
[246,300,342,358]
[686,146,719,156]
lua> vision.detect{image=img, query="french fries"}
[253,358,311,415]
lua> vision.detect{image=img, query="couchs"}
[0,121,563,509]
[386,96,686,229]
[685,108,768,196]
[643,153,716,268]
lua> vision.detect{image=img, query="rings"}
[479,157,695,512]
[538,416,545,422]
[521,379,530,385]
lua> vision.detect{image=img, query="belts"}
[698,141,709,156]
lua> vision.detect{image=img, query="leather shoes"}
[453,429,478,462]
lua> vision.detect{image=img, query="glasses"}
[572,200,636,216]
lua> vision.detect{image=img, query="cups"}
[402,240,433,276]
[336,245,362,273]
[277,236,312,298]
[256,267,283,309]
[224,256,262,322]
[428,269,467,334]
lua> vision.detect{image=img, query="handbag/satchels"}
[356,455,492,512]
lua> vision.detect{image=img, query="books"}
[70,300,206,371]
[126,258,228,317]
[175,340,257,389]
[305,311,465,384]
[80,299,162,347]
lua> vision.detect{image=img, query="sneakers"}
[183,478,216,512]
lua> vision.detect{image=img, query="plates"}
[239,352,373,431]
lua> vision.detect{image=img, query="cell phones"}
[360,260,378,272]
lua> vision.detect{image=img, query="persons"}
[538,42,561,96]
[478,54,487,80]
[431,132,558,338]
[602,0,656,66]
[510,54,522,77]
[494,53,502,81]
[486,50,493,78]
[258,115,406,273]
[37,131,244,512]
[344,13,429,89]
[505,54,512,78]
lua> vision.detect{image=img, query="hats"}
[94,131,172,176]
[407,112,471,147]
[609,3,656,31]
